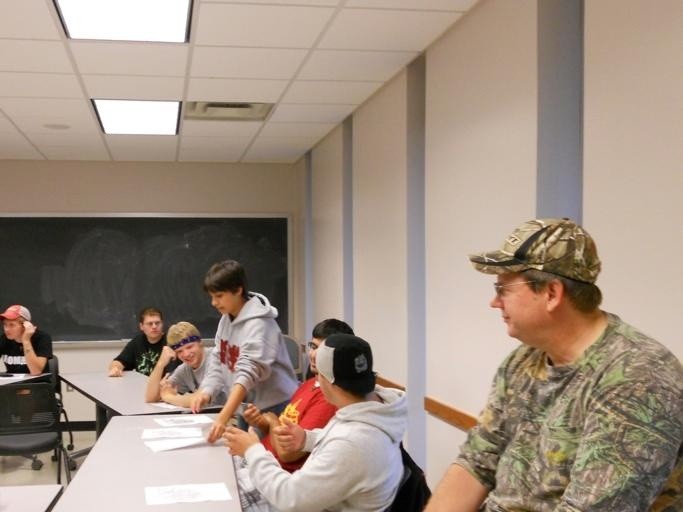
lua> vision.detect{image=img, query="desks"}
[0,484,63,512]
[0,373,53,390]
[49,413,242,512]
[58,369,225,471]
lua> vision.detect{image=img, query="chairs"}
[0,382,58,470]
[48,355,74,451]
[283,334,303,384]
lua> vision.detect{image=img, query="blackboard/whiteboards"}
[0,213,294,348]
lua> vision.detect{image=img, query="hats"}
[468,218,603,285]
[315,333,375,394]
[0,304,32,322]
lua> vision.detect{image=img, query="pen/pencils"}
[0,374,14,378]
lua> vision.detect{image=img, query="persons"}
[223,332,410,512]
[421,219,683,511]
[0,306,52,376]
[108,308,182,377]
[143,319,230,408]
[191,259,300,444]
[240,318,356,473]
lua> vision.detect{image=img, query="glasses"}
[492,277,543,296]
[307,339,320,351]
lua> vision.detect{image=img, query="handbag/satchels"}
[387,473,432,512]
